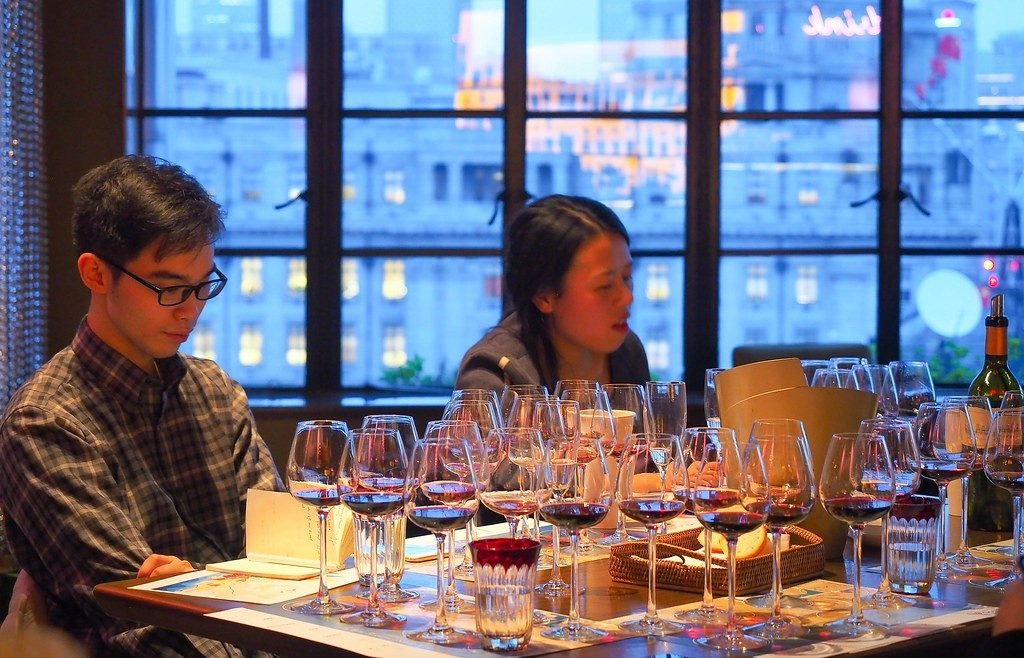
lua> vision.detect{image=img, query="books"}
[207,489,355,580]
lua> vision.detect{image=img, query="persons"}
[455,195,724,527]
[0,155,286,658]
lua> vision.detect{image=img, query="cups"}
[885,495,944,594]
[350,511,404,586]
[468,537,542,652]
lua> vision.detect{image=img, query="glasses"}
[97,255,229,307]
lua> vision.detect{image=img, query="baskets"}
[608,520,823,595]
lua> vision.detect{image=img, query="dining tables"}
[92,513,1024,658]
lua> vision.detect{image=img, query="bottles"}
[968,293,1023,534]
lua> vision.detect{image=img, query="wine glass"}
[738,419,817,641]
[337,428,408,629]
[286,420,357,616]
[818,434,896,643]
[356,415,422,603]
[403,438,478,645]
[696,443,770,651]
[615,433,685,634]
[538,438,612,641]
[419,358,1024,624]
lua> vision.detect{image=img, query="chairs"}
[732,343,874,386]
[0,569,57,635]
